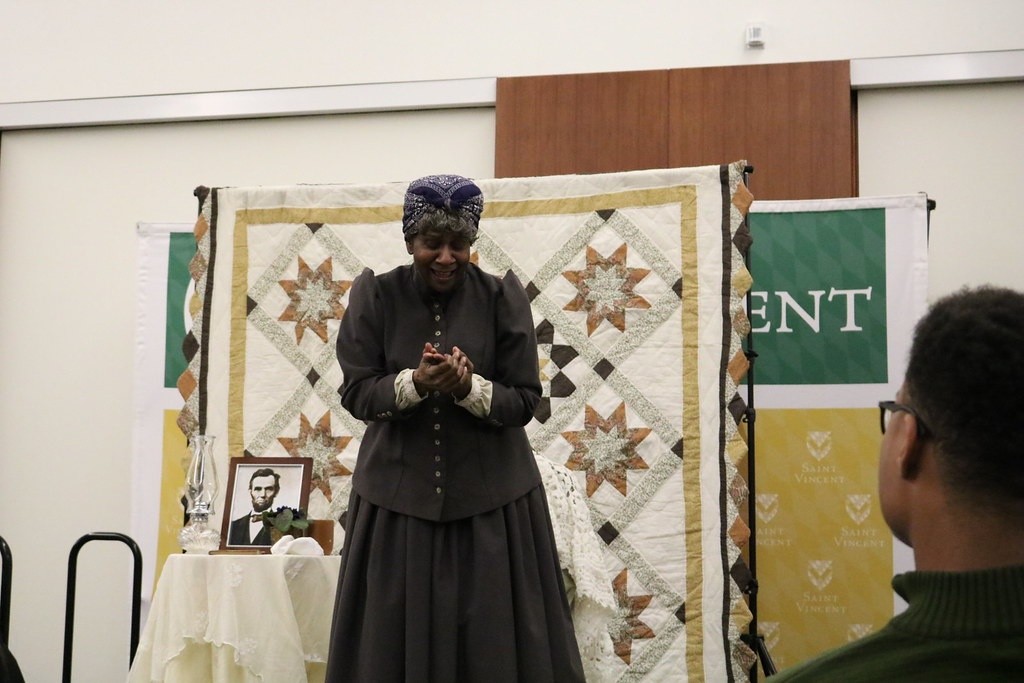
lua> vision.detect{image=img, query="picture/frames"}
[218,456,314,550]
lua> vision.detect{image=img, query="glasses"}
[878,400,927,439]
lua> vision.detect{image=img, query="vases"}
[178,435,219,555]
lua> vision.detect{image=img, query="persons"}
[765,284,1024,683]
[325,174,586,683]
[228,469,280,545]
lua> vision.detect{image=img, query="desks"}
[127,553,344,682]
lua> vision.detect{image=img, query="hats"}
[401,173,483,232]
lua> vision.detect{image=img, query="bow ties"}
[252,514,263,522]
